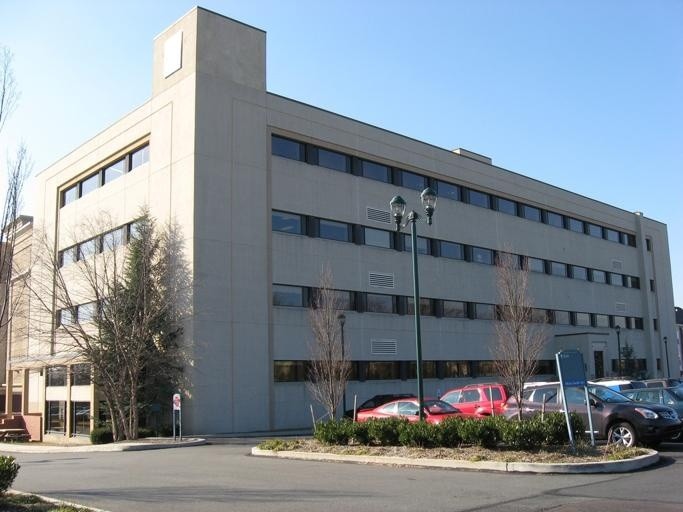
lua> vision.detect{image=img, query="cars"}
[343,393,485,427]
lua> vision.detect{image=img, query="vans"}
[426,383,508,417]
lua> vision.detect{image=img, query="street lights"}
[662,336,670,378]
[388,186,438,421]
[615,325,621,376]
[337,313,347,417]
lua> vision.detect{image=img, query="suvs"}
[504,377,683,449]
